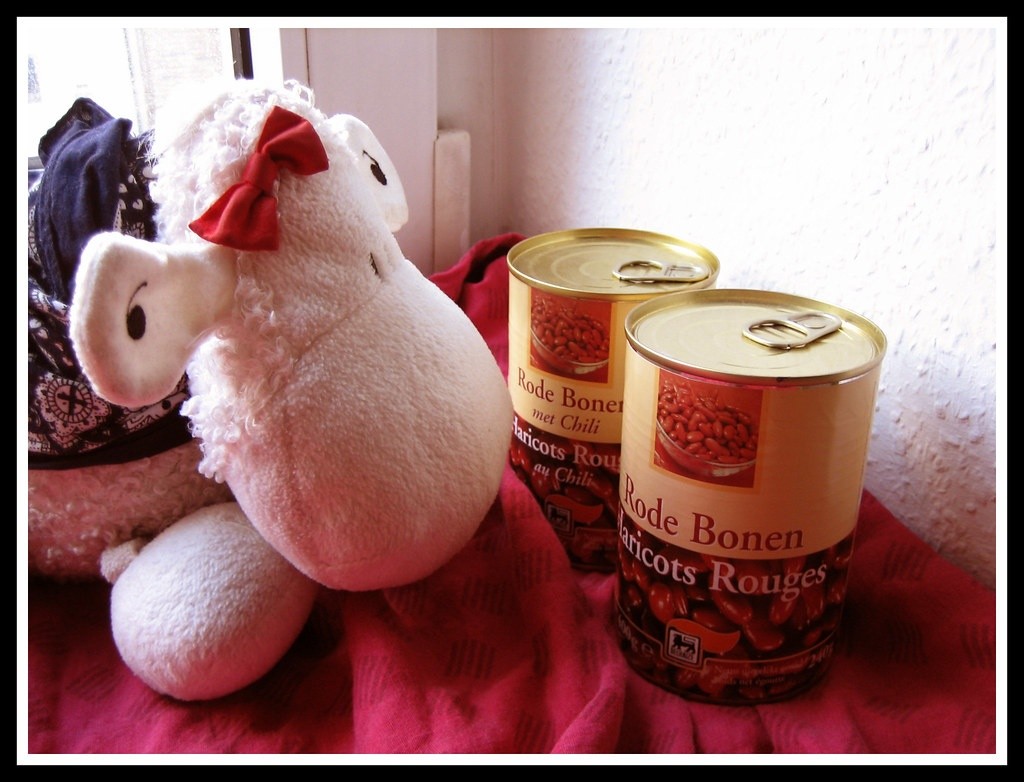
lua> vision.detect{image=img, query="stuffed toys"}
[29,79,515,702]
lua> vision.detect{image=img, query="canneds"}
[609,286,889,701]
[504,227,722,574]
